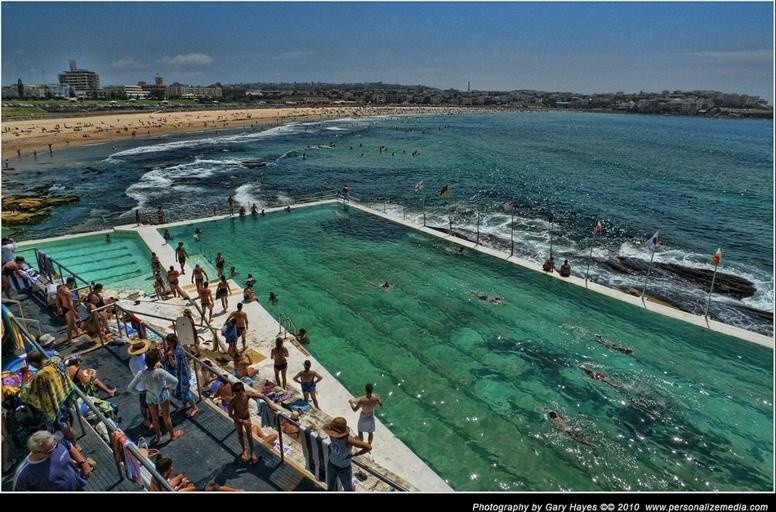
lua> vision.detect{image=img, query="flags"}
[440,185,449,197]
[545,212,554,222]
[644,231,659,251]
[712,249,722,267]
[595,220,602,232]
[467,192,480,204]
[501,200,513,213]
[414,181,423,192]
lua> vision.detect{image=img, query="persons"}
[227,194,265,217]
[271,337,289,390]
[135,209,142,224]
[580,365,623,388]
[164,230,170,237]
[157,205,166,223]
[549,409,599,450]
[593,334,632,354]
[561,260,570,277]
[1,109,553,162]
[106,233,112,241]
[342,185,350,201]
[195,227,201,234]
[1,239,279,494]
[293,360,323,410]
[543,256,555,271]
[470,290,501,304]
[371,276,393,290]
[349,383,382,445]
[291,328,310,344]
[455,246,465,256]
[323,417,371,490]
